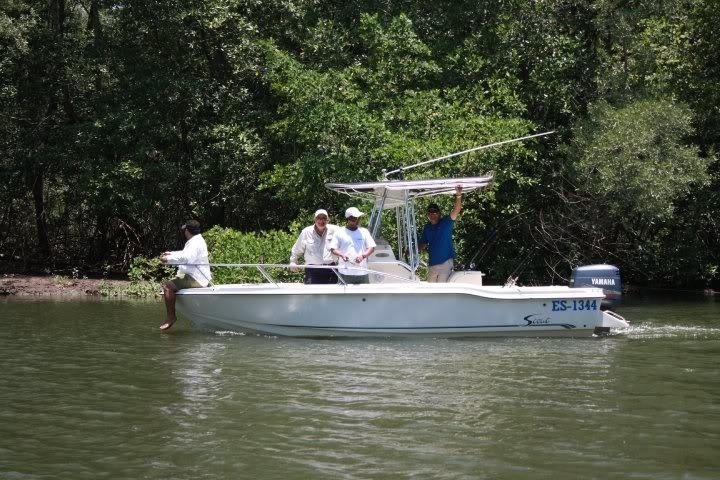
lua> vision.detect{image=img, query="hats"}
[182,223,199,230]
[315,209,328,218]
[345,207,365,219]
[426,203,439,212]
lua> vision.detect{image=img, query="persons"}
[159,220,212,330]
[330,207,377,284]
[405,185,463,283]
[289,209,340,284]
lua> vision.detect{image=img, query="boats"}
[161,129,631,337]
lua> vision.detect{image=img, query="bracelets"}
[362,255,366,258]
[456,195,462,198]
